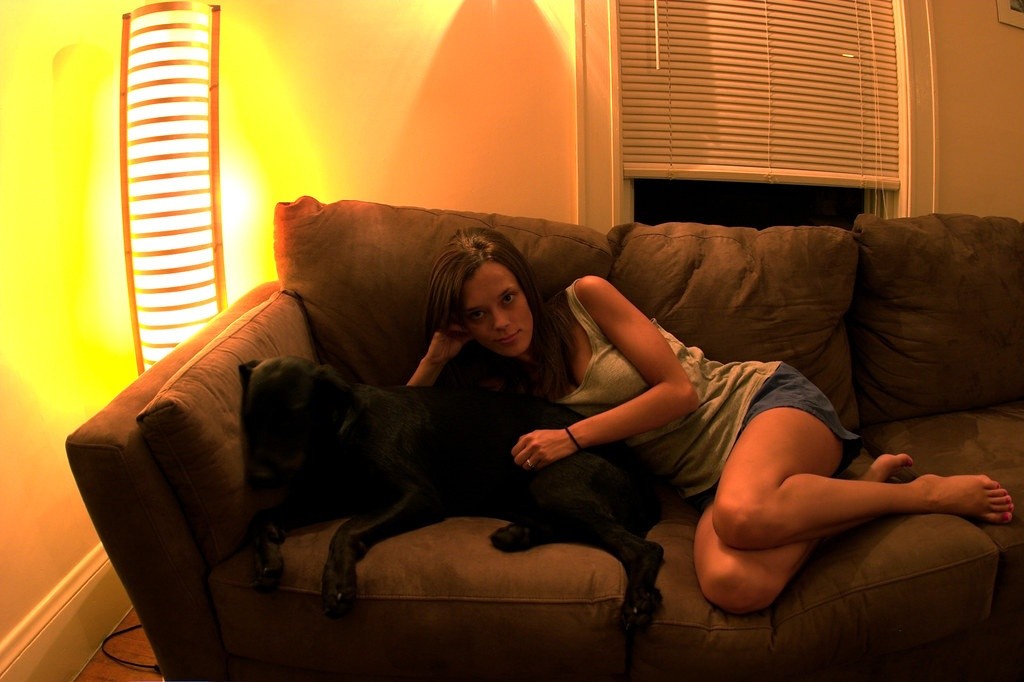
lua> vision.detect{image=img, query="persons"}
[405,226,1014,615]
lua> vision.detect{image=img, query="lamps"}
[120,0,225,379]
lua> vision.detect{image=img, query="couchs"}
[67,195,1024,682]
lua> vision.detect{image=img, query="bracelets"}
[564,425,582,451]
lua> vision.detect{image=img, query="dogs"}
[237,355,683,632]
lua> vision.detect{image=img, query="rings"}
[526,460,536,469]
